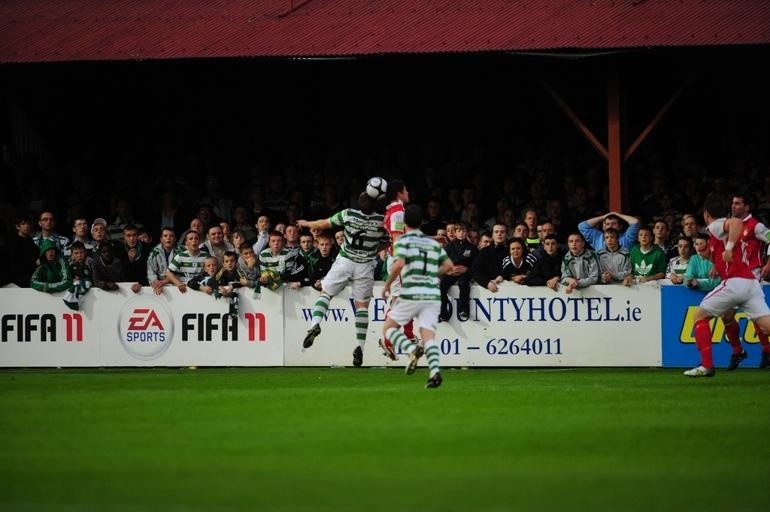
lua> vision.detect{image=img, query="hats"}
[91,218,107,233]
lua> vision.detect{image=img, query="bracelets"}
[726,242,734,251]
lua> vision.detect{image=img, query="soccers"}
[367,176,388,199]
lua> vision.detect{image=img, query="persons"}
[382,204,453,389]
[296,192,391,368]
[379,252,419,359]
[0,156,769,389]
[682,199,770,378]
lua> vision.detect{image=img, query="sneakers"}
[405,346,441,388]
[684,351,769,377]
[303,323,320,348]
[353,346,362,365]
